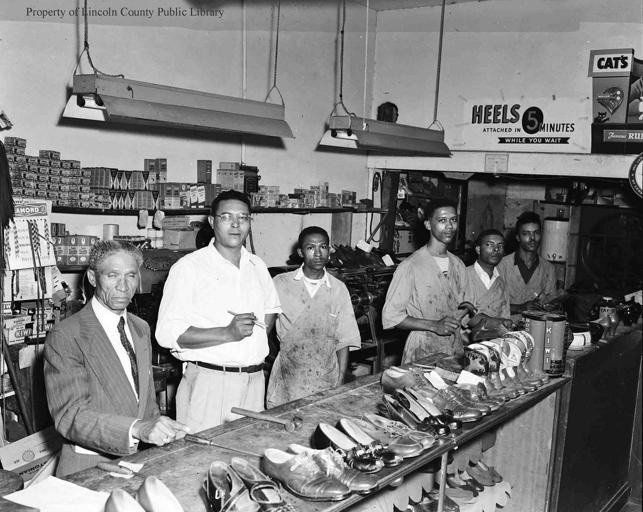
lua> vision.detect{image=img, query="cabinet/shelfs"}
[52,204,402,415]
[0,356,566,512]
[376,171,461,260]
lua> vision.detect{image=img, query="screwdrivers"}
[185,434,264,459]
[97,462,147,478]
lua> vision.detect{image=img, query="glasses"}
[215,213,251,223]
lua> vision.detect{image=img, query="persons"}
[263,227,361,408]
[44,238,188,480]
[380,197,471,365]
[154,190,282,437]
[502,210,557,323]
[463,230,511,325]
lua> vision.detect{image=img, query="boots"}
[463,310,567,402]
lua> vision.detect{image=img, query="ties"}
[116,316,140,400]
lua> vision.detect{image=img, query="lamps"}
[317,0,456,159]
[55,1,295,142]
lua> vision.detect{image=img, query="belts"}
[190,360,265,374]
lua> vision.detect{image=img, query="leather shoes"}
[378,361,505,440]
[288,444,379,496]
[309,412,435,475]
[261,448,351,502]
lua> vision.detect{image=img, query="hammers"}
[456,301,478,320]
[232,407,304,432]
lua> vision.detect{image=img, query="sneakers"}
[567,295,641,351]
[400,459,504,512]
[202,460,263,512]
[230,456,295,512]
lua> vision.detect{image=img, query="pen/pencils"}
[228,310,265,330]
